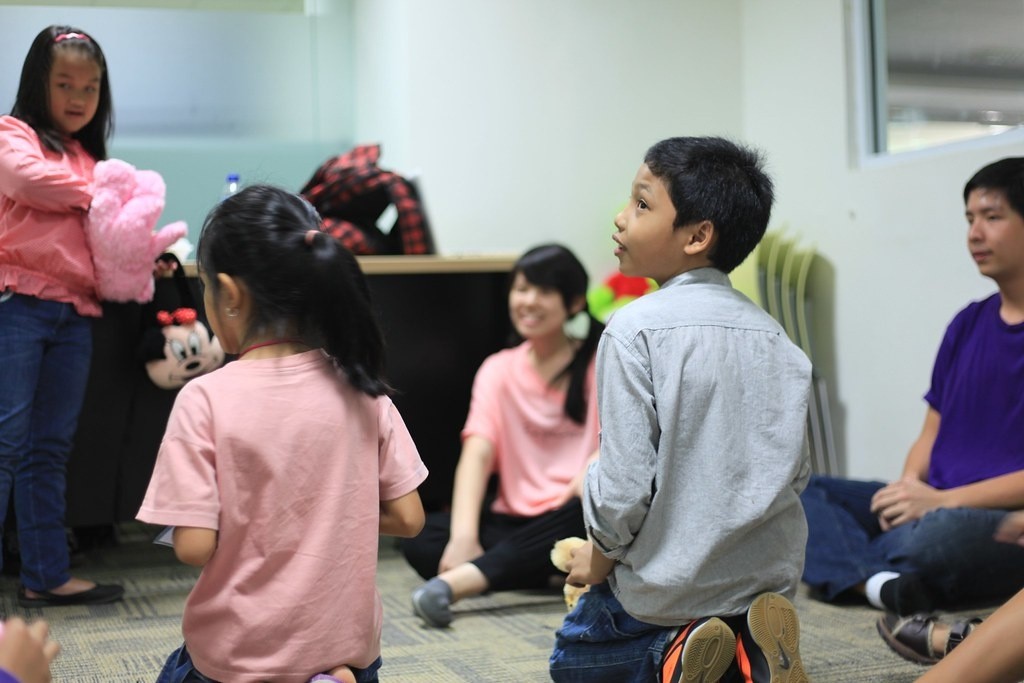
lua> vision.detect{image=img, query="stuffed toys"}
[87,158,189,307]
[135,252,227,392]
[550,535,594,612]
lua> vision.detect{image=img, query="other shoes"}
[413,577,453,627]
[880,572,940,615]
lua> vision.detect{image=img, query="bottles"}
[219,174,242,201]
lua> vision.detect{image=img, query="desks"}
[64,256,523,549]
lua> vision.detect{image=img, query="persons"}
[548,134,819,683]
[131,183,433,683]
[800,153,1023,616]
[2,21,127,608]
[875,586,1024,683]
[0,616,62,683]
[393,244,608,630]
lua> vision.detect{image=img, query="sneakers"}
[659,616,737,683]
[736,591,811,683]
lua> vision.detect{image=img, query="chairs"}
[728,236,841,478]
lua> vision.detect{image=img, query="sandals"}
[18,581,124,607]
[877,613,983,665]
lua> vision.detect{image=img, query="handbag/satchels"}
[299,145,435,256]
